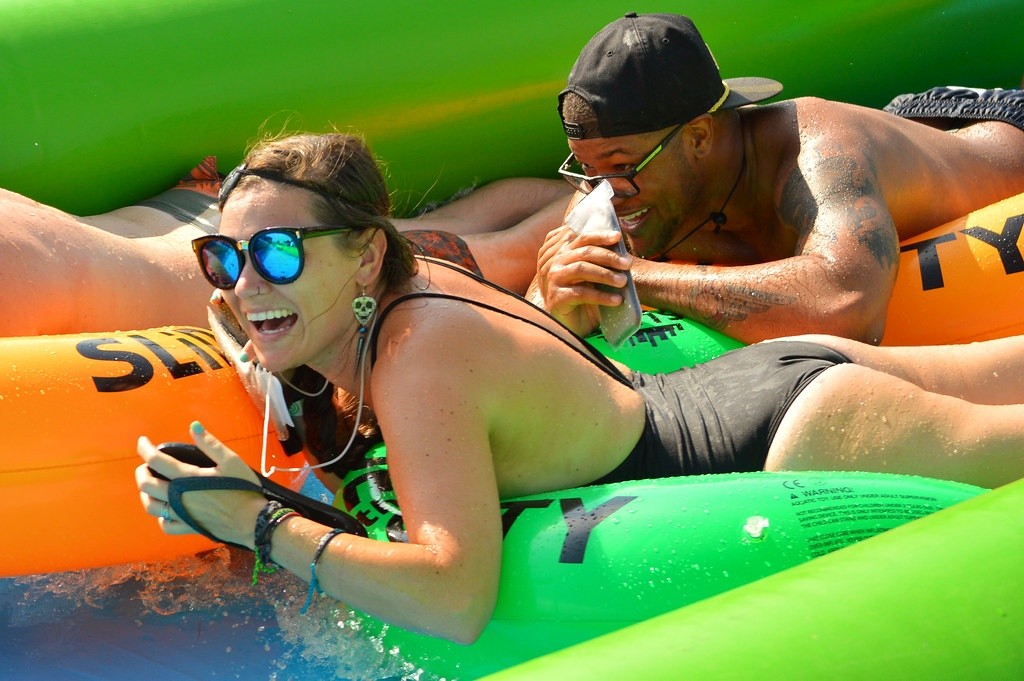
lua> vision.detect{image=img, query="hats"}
[556,11,783,141]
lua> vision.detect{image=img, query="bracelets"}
[300,528,346,612]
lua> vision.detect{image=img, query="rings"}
[162,502,168,520]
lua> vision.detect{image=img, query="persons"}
[555,12,1024,346]
[134,132,1024,646]
[248,501,302,585]
[0,154,578,338]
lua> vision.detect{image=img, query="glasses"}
[191,223,354,291]
[558,122,690,199]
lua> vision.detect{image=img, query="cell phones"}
[580,209,643,345]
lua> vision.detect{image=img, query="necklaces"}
[652,154,747,265]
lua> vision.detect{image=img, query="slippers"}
[148,441,369,572]
